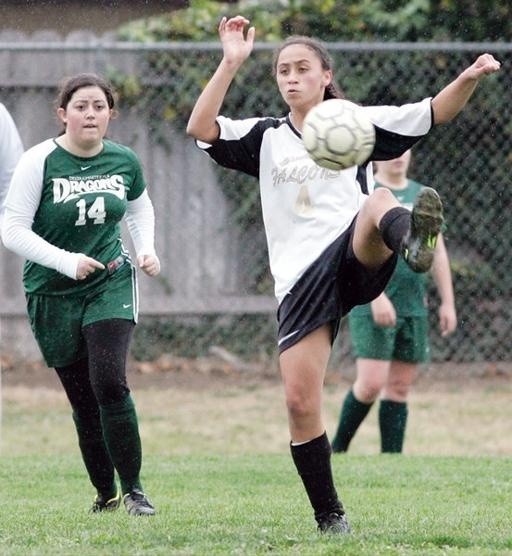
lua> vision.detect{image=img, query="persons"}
[2,73,163,516]
[184,15,502,538]
[330,147,458,455]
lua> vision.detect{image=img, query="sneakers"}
[91,489,120,513]
[401,188,444,275]
[122,488,155,518]
[317,509,350,534]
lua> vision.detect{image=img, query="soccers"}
[302,98,377,169]
